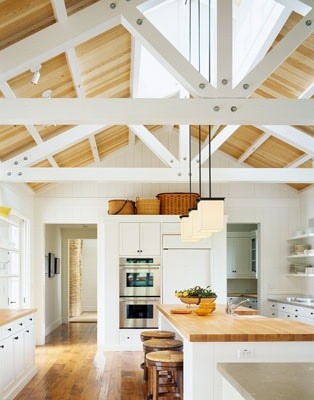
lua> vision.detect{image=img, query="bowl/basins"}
[195,308,211,315]
[199,303,216,314]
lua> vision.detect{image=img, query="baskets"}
[157,192,201,216]
[107,200,137,215]
[136,198,161,215]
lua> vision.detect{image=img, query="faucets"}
[226,298,250,315]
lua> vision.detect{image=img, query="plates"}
[171,309,192,313]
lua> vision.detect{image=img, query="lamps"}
[30,64,41,85]
[179,125,225,243]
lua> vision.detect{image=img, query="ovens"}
[119,256,161,329]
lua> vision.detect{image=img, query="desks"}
[155,305,314,400]
[217,362,314,400]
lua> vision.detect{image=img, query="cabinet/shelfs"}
[227,297,257,309]
[96,215,227,351]
[250,230,258,279]
[0,312,37,400]
[285,233,314,277]
[227,232,250,279]
[267,300,314,325]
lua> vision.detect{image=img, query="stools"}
[141,339,182,383]
[145,350,183,400]
[140,330,176,369]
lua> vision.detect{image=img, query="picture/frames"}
[48,253,55,277]
[55,258,60,274]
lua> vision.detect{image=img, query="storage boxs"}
[108,192,200,215]
